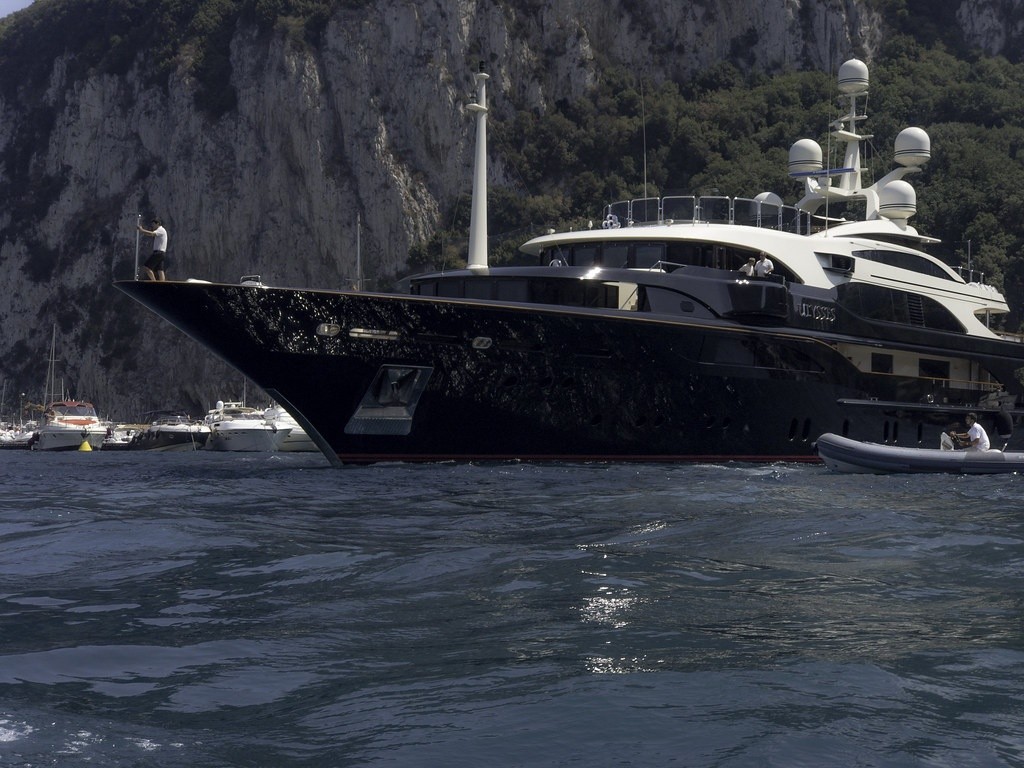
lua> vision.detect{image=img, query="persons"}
[738,251,775,281]
[127,429,130,436]
[2,424,21,433]
[950,412,990,452]
[136,219,168,281]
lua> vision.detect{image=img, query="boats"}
[0,322,323,452]
[110,54,1024,469]
[816,431,1024,474]
